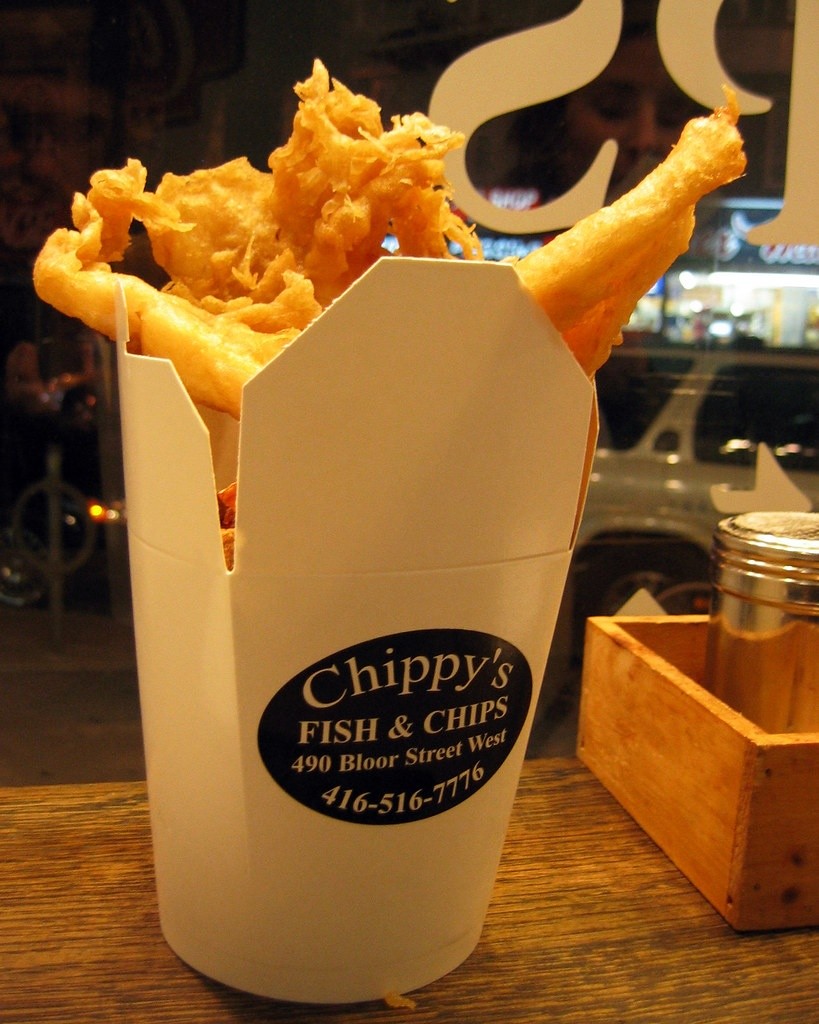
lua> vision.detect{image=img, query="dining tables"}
[2,756,819,1024]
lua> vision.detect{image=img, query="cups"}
[706,505,819,735]
[775,284,811,346]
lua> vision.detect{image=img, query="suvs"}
[556,331,819,664]
